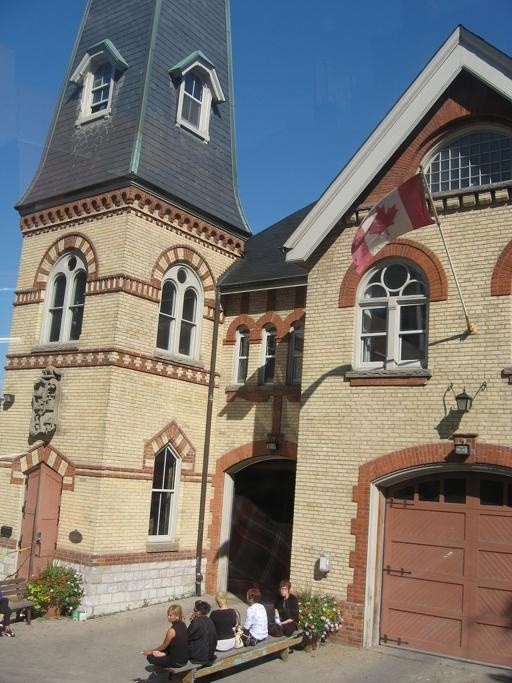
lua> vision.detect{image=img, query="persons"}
[242,589,268,642]
[187,600,217,660]
[140,605,188,668]
[268,580,299,637]
[209,591,237,651]
[0,591,15,637]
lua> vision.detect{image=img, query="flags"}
[351,173,435,275]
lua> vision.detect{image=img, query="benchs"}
[0,578,34,625]
[162,631,303,683]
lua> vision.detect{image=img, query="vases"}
[44,598,61,617]
[305,636,319,650]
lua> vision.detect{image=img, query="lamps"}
[455,441,470,456]
[448,381,487,411]
[319,553,331,578]
[266,437,279,450]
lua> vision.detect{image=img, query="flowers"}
[293,579,344,639]
[26,558,85,612]
